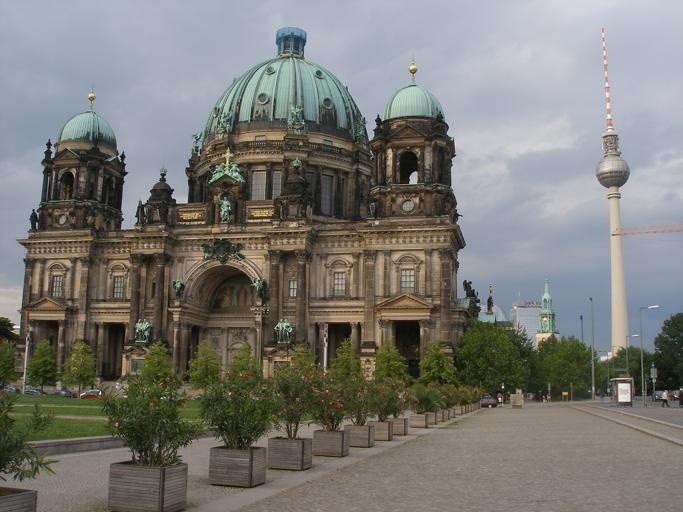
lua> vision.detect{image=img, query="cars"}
[117,386,260,401]
[479,396,496,408]
[652,389,682,408]
[0,384,102,400]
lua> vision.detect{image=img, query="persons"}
[141,318,152,342]
[218,195,232,222]
[282,318,292,342]
[274,318,284,343]
[134,318,142,340]
[496,390,503,407]
[661,389,670,408]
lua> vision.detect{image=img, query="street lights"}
[22,334,31,397]
[579,315,584,345]
[588,297,596,401]
[599,303,660,411]
